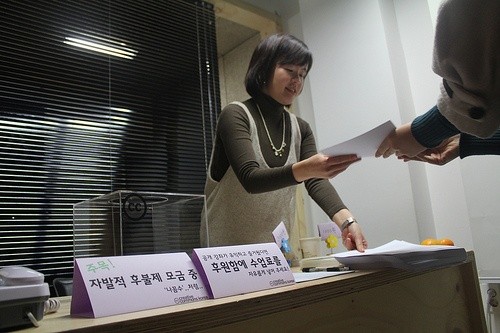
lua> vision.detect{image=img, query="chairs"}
[53,277,73,297]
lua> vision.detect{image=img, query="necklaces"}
[251,96,289,157]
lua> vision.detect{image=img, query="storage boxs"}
[73,190,209,260]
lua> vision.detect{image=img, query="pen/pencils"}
[302,267,351,272]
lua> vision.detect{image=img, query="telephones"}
[0,266,61,333]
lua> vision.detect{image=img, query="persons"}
[200,31,368,263]
[374,0,500,165]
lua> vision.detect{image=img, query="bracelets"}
[339,216,357,232]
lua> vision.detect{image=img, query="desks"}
[0,249,489,333]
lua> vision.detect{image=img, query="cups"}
[299,237,322,259]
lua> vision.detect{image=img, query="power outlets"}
[488,283,500,309]
